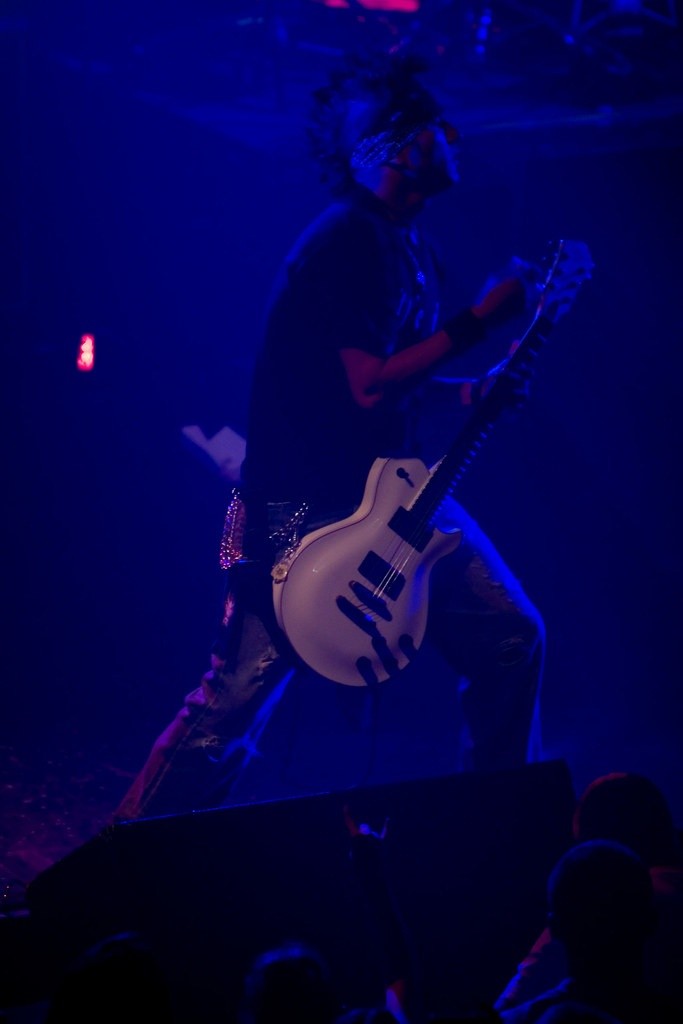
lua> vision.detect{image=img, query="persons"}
[495,770,683,1010]
[101,46,546,833]
[241,943,337,1024]
[499,840,682,1022]
[43,945,170,1023]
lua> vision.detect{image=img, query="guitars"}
[270,237,598,692]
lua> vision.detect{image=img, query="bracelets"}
[442,307,488,352]
